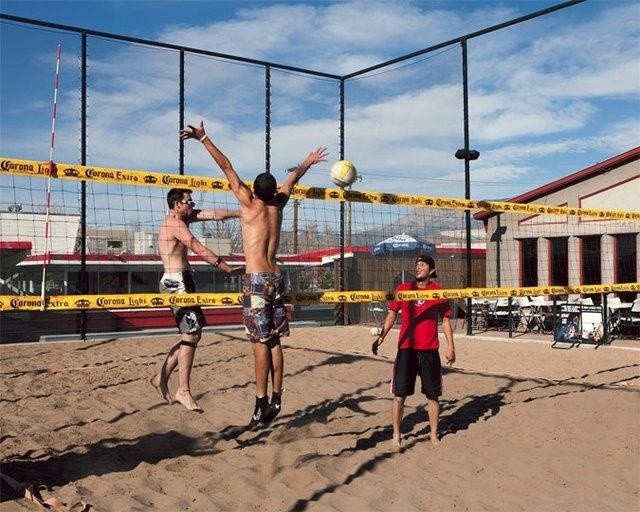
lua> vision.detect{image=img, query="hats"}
[254,173,276,202]
[416,256,437,278]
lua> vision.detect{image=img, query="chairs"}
[461,296,640,339]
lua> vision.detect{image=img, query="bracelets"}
[213,258,222,267]
[199,134,207,142]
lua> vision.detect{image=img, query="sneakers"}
[248,392,282,431]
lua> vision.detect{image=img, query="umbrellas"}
[372,232,437,284]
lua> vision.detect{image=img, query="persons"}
[179,119,330,431]
[148,187,243,414]
[371,254,456,446]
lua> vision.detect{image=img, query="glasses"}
[177,200,195,206]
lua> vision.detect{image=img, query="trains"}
[15,250,330,339]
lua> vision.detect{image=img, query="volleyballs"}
[330,158,357,187]
